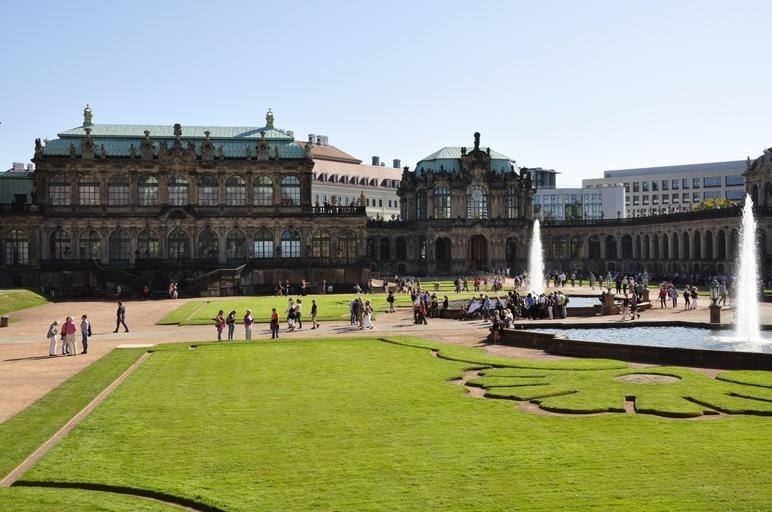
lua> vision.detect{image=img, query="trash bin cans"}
[434,283,440,290]
[0,316,9,327]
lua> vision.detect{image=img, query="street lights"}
[707,278,722,324]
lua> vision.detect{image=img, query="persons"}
[226,310,236,340]
[216,309,226,340]
[47,280,179,356]
[244,309,254,340]
